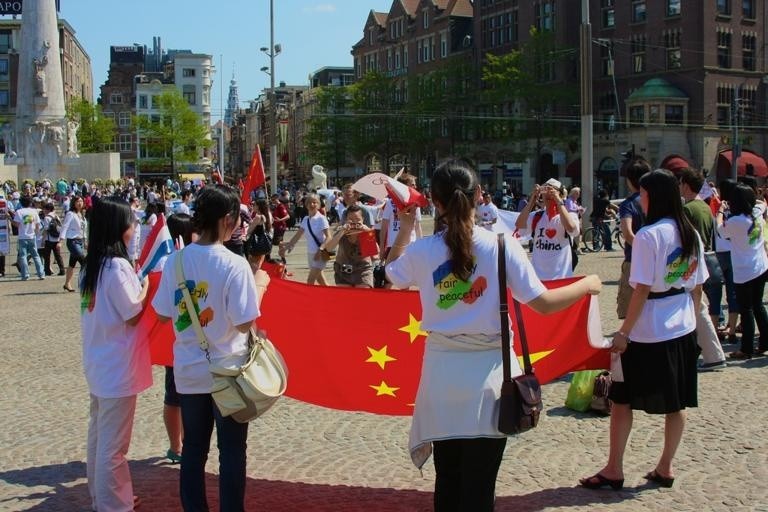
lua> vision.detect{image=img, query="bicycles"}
[583,213,626,252]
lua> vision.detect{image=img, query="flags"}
[148,272,623,416]
[241,144,266,204]
[136,213,175,282]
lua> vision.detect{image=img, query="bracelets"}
[526,205,532,212]
[319,247,324,251]
[391,242,411,248]
[718,211,724,215]
[256,284,267,291]
[617,331,630,343]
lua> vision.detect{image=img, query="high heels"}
[166,449,184,467]
[62,285,75,293]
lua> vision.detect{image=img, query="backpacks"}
[531,210,578,271]
[46,214,62,238]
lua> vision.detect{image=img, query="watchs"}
[558,200,565,207]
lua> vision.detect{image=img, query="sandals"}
[730,351,753,359]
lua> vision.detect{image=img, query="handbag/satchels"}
[497,235,542,435]
[703,215,726,286]
[592,371,612,415]
[176,254,290,423]
[308,220,338,256]
[565,369,598,412]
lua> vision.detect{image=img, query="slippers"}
[580,472,628,492]
[642,468,674,486]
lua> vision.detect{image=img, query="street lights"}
[259,0,282,196]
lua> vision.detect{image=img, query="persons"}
[560,187,568,200]
[263,176,271,199]
[580,169,709,490]
[589,189,620,252]
[278,193,331,285]
[479,192,498,231]
[617,159,652,319]
[5,180,51,216]
[518,194,528,212]
[13,196,45,281]
[515,178,580,281]
[319,191,343,225]
[44,203,66,276]
[384,159,601,512]
[249,199,271,269]
[126,197,141,264]
[716,185,768,359]
[332,182,370,229]
[680,169,726,372]
[365,197,385,269]
[299,192,308,222]
[271,194,290,275]
[166,176,205,191]
[709,182,720,199]
[256,186,265,199]
[311,189,317,194]
[566,187,586,255]
[279,190,289,206]
[174,191,192,216]
[56,196,85,292]
[36,211,45,259]
[151,185,271,512]
[79,196,149,511]
[375,202,386,224]
[736,175,759,333]
[480,190,485,205]
[0,200,11,276]
[224,185,253,257]
[140,203,165,255]
[57,177,163,214]
[295,191,303,223]
[80,209,87,249]
[703,195,720,343]
[380,175,422,290]
[494,184,513,211]
[164,212,199,463]
[713,180,742,344]
[325,205,380,289]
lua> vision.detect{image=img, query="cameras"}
[350,222,361,229]
[538,185,549,194]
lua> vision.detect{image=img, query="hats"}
[668,157,688,171]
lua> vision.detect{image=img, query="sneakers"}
[697,360,727,371]
[135,494,145,508]
